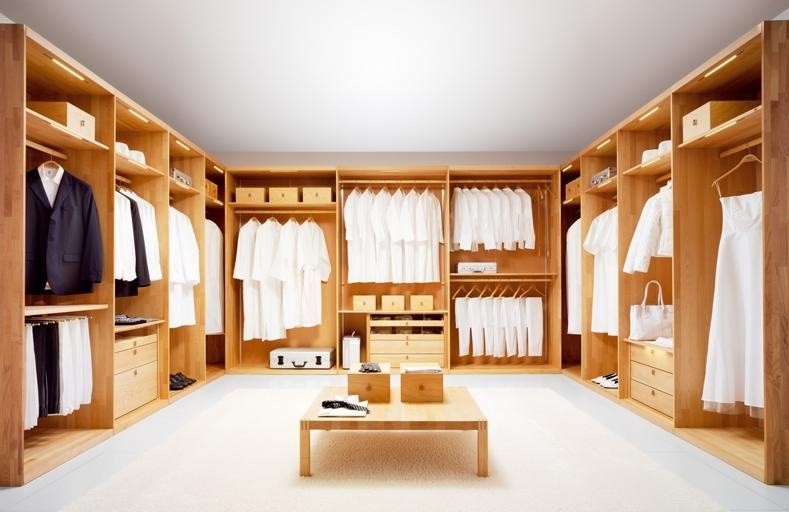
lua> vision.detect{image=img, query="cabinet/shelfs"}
[1,23,114,487]
[337,166,447,372]
[225,168,338,372]
[114,87,167,434]
[672,21,788,486]
[559,154,580,384]
[204,152,228,381]
[167,125,206,399]
[579,128,618,401]
[618,90,673,434]
[448,166,564,373]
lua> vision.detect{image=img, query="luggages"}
[171,167,194,186]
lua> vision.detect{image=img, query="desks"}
[298,385,491,478]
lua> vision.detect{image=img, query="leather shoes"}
[170,372,197,390]
[591,371,619,389]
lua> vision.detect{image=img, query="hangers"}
[114,175,134,194]
[38,152,61,169]
[248,212,317,226]
[608,196,618,211]
[24,313,96,329]
[450,277,546,302]
[709,143,762,188]
[351,182,438,196]
[453,181,558,201]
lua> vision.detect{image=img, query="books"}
[405,364,441,373]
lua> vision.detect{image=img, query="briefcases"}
[591,167,616,186]
[457,260,498,275]
[269,346,335,369]
[342,330,361,370]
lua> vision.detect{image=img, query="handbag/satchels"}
[629,280,674,341]
